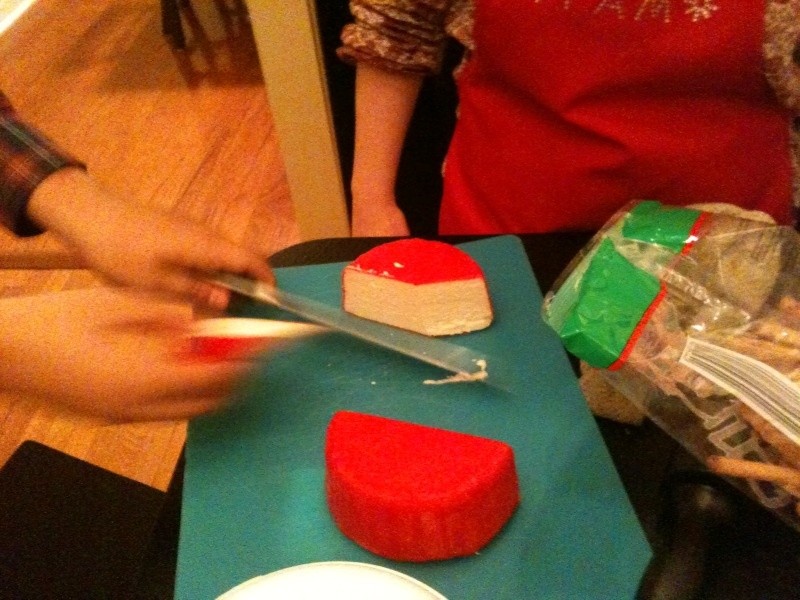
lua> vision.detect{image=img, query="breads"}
[340,238,496,337]
[324,411,522,561]
[187,320,328,362]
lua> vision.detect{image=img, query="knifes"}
[191,266,492,383]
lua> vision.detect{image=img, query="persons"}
[0,91,277,424]
[336,0,800,236]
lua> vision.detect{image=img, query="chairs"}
[248,1,459,241]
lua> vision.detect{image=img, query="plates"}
[215,560,451,600]
